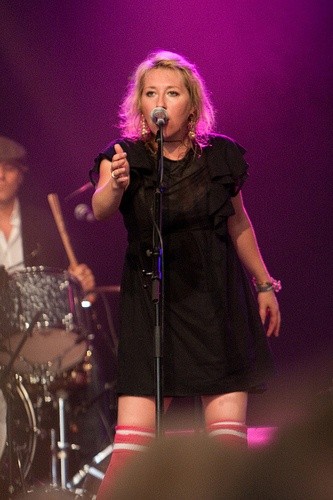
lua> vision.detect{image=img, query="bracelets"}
[253,279,280,292]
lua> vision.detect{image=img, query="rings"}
[112,171,120,178]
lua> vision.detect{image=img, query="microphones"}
[151,107,168,127]
[74,204,95,222]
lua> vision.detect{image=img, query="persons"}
[86,52,281,499]
[0,139,95,300]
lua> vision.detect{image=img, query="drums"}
[0,377,37,500]
[0,266,89,377]
